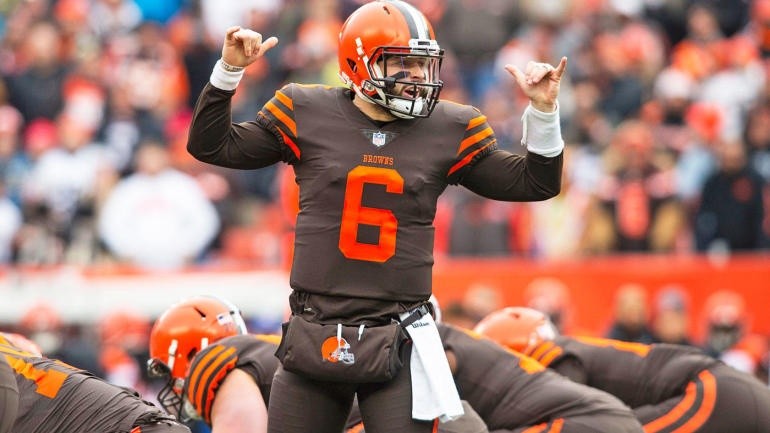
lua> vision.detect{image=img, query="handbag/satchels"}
[274,302,428,384]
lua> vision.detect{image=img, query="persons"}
[0,0,770,271]
[0,337,191,433]
[431,321,643,433]
[145,296,365,433]
[474,307,770,433]
[185,0,567,433]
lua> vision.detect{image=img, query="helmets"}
[474,307,556,356]
[337,0,445,118]
[146,297,248,422]
